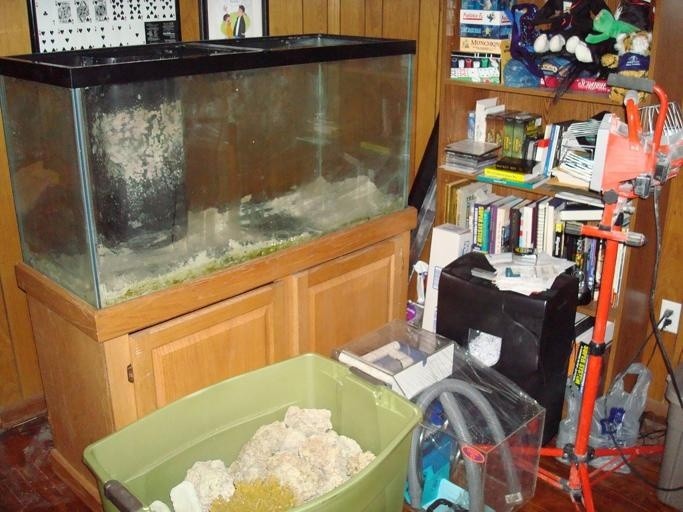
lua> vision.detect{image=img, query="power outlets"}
[658,298,682,334]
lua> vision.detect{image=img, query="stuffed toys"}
[504,0,653,106]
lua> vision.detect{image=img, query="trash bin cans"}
[654,366,683,510]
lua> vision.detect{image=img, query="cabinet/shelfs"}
[436,0,683,398]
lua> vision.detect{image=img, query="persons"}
[221,12,233,39]
[232,4,246,38]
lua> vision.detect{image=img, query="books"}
[453,182,609,302]
[445,97,595,191]
[563,312,615,400]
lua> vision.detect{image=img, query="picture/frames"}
[26,0,181,55]
[199,0,269,41]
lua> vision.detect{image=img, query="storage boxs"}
[83,352,422,511]
[659,362,683,512]
